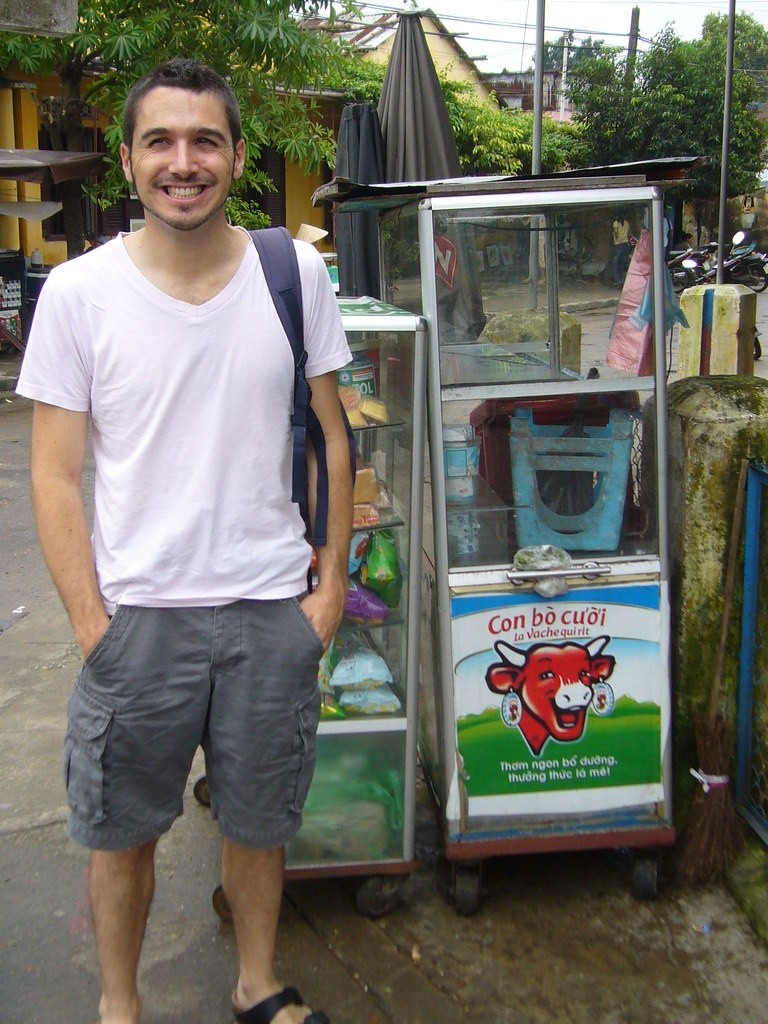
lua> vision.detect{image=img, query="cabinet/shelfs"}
[195,185,677,927]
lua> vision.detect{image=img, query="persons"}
[13,64,355,1024]
[679,232,693,250]
[612,210,630,284]
[663,215,672,262]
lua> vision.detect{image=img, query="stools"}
[507,406,635,553]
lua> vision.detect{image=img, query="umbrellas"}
[0,149,111,183]
[374,10,486,343]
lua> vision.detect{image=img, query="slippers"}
[231,983,329,1024]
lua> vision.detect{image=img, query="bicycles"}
[557,236,592,265]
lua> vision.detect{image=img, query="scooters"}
[665,231,768,296]
